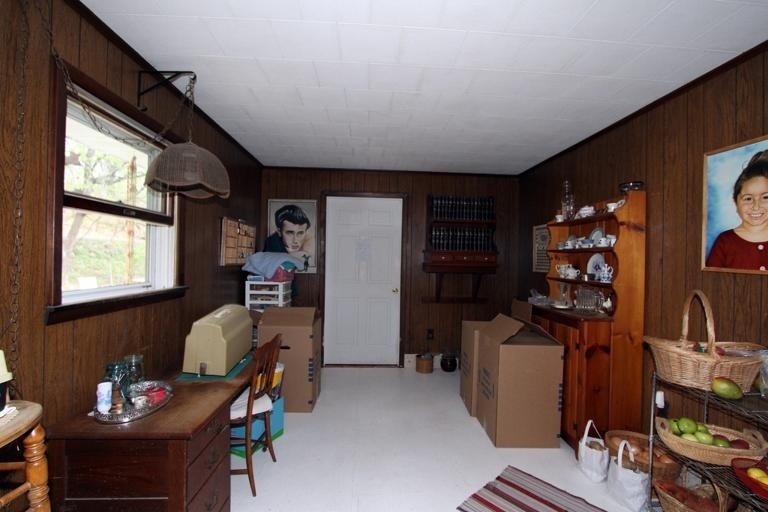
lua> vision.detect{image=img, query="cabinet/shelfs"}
[245,281,291,348]
[636,368,768,512]
[532,191,647,453]
[45,379,231,511]
[422,194,500,303]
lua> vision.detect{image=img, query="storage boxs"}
[459,320,492,416]
[258,306,321,413]
[479,313,565,449]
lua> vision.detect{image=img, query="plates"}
[731,457,768,501]
[589,228,603,244]
[586,253,606,280]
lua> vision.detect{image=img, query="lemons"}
[747,466,768,485]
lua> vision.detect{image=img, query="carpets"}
[456,465,607,512]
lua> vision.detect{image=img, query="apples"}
[671,417,749,450]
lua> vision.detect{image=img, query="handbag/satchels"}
[578,420,609,483]
[607,439,650,512]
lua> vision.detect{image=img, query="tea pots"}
[555,263,580,279]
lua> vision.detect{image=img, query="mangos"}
[711,376,745,400]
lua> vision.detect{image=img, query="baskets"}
[643,289,766,392]
[607,416,768,512]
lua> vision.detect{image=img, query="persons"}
[263,204,315,268]
[705,149,768,272]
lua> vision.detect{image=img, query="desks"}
[0,400,51,512]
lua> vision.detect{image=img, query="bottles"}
[561,180,575,221]
[103,354,145,397]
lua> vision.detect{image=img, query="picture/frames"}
[264,198,319,275]
[699,134,767,275]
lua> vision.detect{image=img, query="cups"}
[576,290,603,315]
[132,396,147,410]
[97,382,112,414]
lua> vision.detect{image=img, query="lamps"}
[131,69,233,203]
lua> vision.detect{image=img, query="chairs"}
[231,333,285,498]
